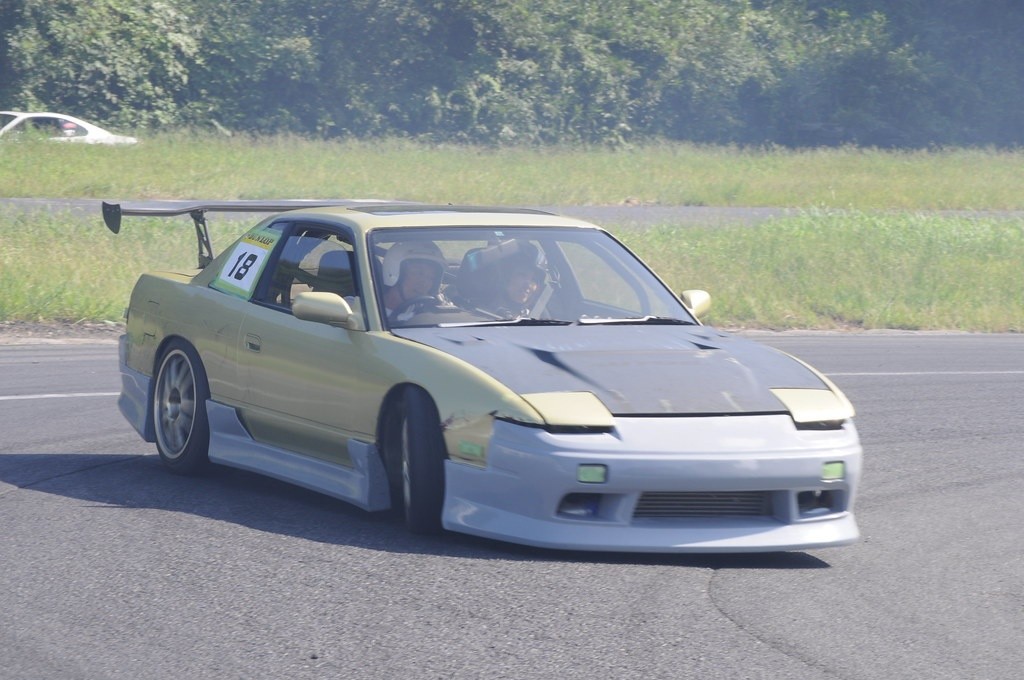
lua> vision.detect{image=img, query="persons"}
[343,239,446,327]
[470,237,556,320]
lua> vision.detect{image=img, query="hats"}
[515,243,548,282]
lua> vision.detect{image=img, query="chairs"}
[313,250,384,304]
[447,247,501,309]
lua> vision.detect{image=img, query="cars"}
[0,110,137,145]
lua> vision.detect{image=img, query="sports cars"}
[95,193,863,555]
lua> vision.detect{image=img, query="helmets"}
[383,240,444,298]
